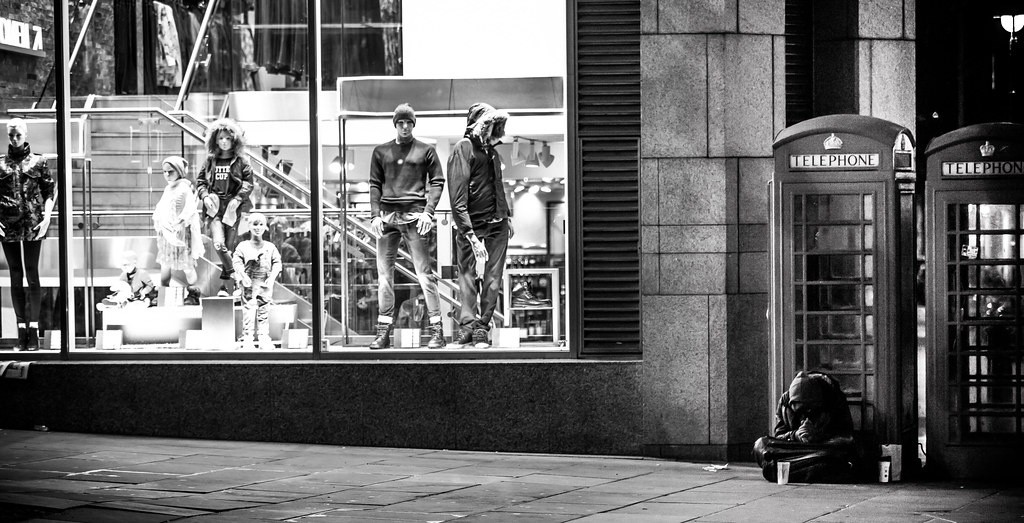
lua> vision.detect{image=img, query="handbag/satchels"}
[762,441,864,485]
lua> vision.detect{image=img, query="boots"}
[185,286,199,304]
[513,287,550,308]
[15,328,27,350]
[27,328,38,350]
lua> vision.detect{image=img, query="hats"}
[393,104,415,127]
[163,156,188,178]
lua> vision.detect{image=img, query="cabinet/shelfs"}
[502,266,561,343]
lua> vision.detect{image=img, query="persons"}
[447,104,514,344]
[117,250,158,306]
[753,371,856,484]
[369,103,446,348]
[0,118,57,351]
[232,213,282,350]
[196,118,253,296]
[152,156,201,305]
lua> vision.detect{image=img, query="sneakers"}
[233,281,242,297]
[446,329,473,349]
[426,322,445,348]
[472,329,489,348]
[216,285,228,297]
[369,323,392,348]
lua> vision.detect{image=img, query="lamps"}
[525,141,540,168]
[537,141,555,168]
[510,137,526,167]
[344,147,354,170]
[329,155,343,173]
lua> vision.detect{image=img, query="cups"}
[879,462,890,482]
[777,462,790,485]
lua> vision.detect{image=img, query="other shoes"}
[244,337,257,350]
[259,334,274,350]
